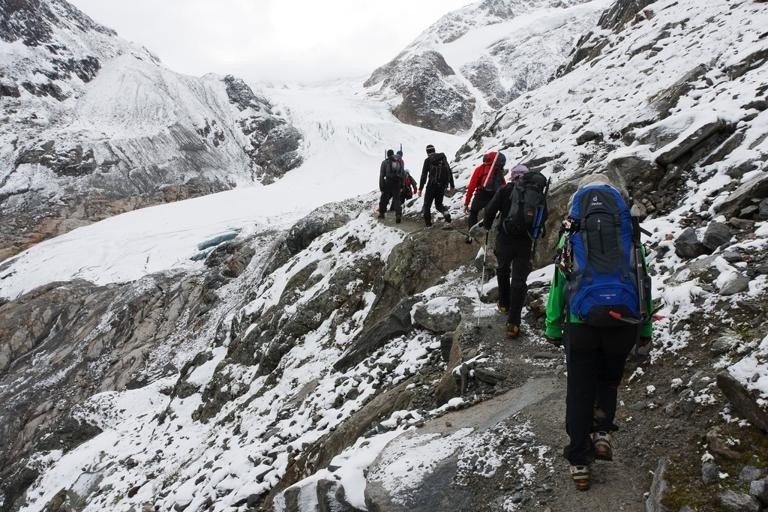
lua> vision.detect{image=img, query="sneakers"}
[507,324,518,337]
[569,465,591,491]
[396,217,401,223]
[499,306,510,315]
[592,432,613,461]
[445,217,451,223]
[426,222,432,227]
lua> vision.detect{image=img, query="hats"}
[387,150,393,155]
[577,173,611,189]
[426,145,435,154]
[512,165,528,180]
[483,154,488,162]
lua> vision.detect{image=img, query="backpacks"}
[384,154,401,185]
[503,171,547,236]
[482,151,505,189]
[559,184,650,330]
[430,153,449,187]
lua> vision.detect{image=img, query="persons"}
[419,144,454,230]
[544,181,655,491]
[482,164,549,337]
[465,151,507,244]
[378,150,417,223]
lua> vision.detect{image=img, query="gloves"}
[483,222,492,230]
[545,333,562,347]
[414,187,417,193]
[638,337,650,347]
